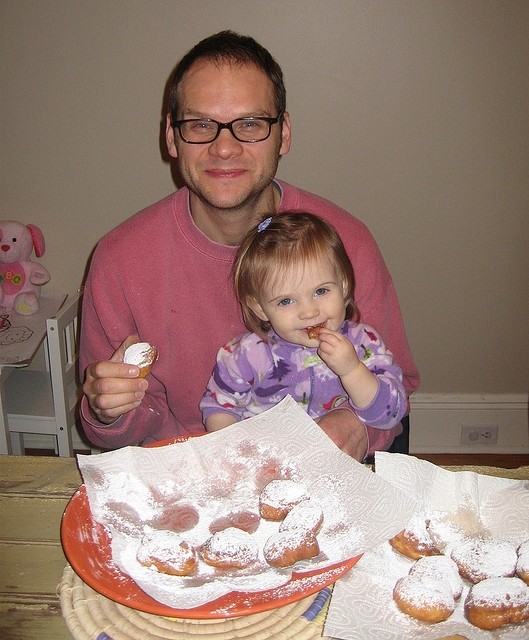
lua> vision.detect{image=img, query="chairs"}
[3,285,89,456]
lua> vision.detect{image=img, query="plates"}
[59,432,366,619]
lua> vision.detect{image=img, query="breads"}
[451,538,518,584]
[199,526,260,570]
[259,479,310,522]
[389,517,441,561]
[392,554,464,624]
[278,498,324,537]
[515,538,529,588]
[122,342,158,379]
[263,527,319,569]
[136,530,198,576]
[434,634,469,640]
[464,576,529,631]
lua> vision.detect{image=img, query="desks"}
[0,290,65,369]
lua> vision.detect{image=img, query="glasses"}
[171,108,283,143]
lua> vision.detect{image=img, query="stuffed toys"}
[0,220,51,315]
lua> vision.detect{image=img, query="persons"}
[199,206,408,432]
[78,30,421,462]
